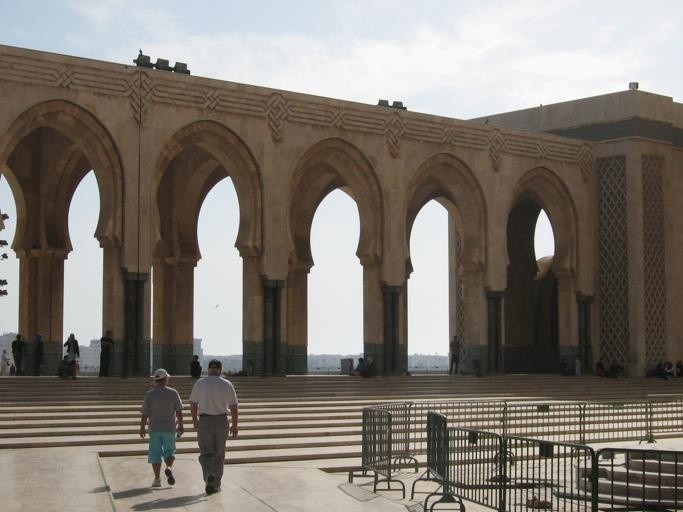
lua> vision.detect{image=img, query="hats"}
[154,369,170,380]
[208,359,222,369]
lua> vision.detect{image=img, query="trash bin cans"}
[341,359,355,375]
[676,362,682,377]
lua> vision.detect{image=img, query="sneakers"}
[205,474,215,493]
[165,467,175,484]
[152,479,161,487]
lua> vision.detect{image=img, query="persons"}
[189,355,202,379]
[62,333,79,375]
[98,330,113,378]
[188,359,239,494]
[609,359,623,378]
[58,355,80,381]
[594,357,607,376]
[222,359,254,377]
[360,356,375,379]
[8,363,15,376]
[10,335,26,376]
[349,357,365,378]
[560,359,572,376]
[0,349,10,376]
[644,360,683,382]
[30,334,44,376]
[572,355,582,376]
[448,335,461,374]
[139,368,184,487]
